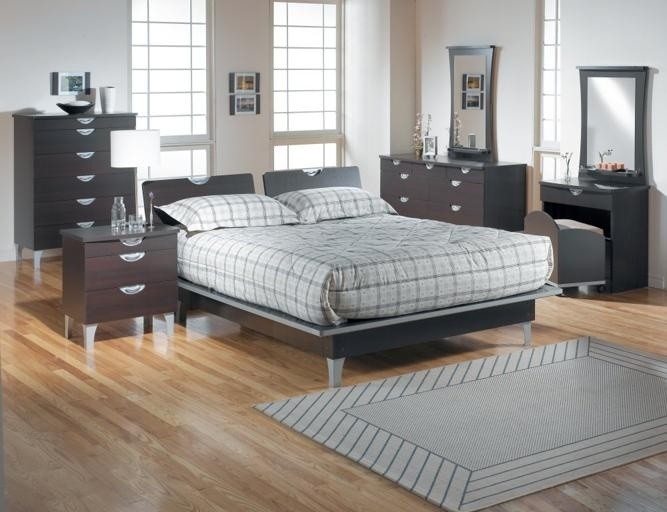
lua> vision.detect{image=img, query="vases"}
[104,86,115,113]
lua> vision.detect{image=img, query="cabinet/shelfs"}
[380,154,527,231]
[11,112,138,268]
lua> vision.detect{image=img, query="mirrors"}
[577,65,649,185]
[445,45,496,163]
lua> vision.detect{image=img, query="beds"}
[141,166,564,388]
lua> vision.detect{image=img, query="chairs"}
[523,210,606,295]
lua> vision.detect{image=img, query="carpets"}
[250,334,667,512]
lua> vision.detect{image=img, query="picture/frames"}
[462,93,484,110]
[229,72,260,93]
[463,74,484,91]
[52,72,91,96]
[467,134,476,148]
[423,136,436,156]
[230,94,261,115]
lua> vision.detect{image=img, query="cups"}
[128,213,144,235]
[99,85,116,115]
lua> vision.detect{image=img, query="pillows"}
[273,187,399,224]
[153,193,300,232]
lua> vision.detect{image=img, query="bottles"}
[110,195,125,233]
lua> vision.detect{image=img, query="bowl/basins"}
[56,99,96,115]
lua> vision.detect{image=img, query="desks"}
[539,177,651,296]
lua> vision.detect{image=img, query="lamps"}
[110,129,160,226]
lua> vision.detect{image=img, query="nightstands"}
[58,222,181,353]
